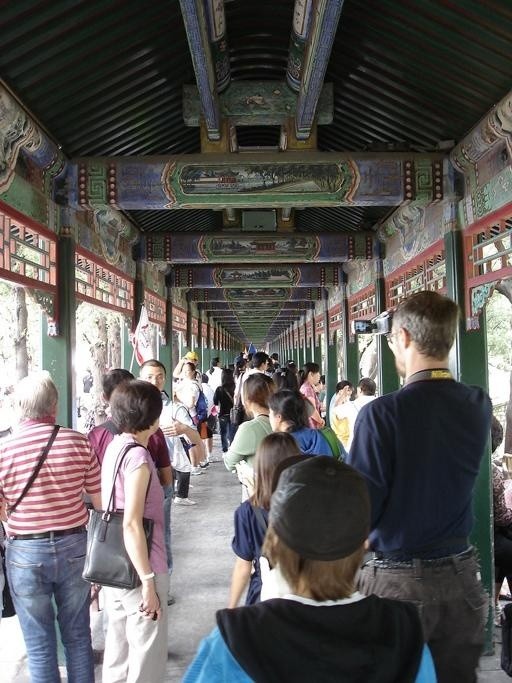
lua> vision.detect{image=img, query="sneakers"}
[174,497,195,505]
[191,456,213,474]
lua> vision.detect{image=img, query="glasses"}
[385,333,401,343]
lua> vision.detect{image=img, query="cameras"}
[351,310,392,336]
[140,603,158,621]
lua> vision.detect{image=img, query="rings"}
[142,610,148,615]
[149,611,155,614]
[139,606,144,612]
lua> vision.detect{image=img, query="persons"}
[350,288,494,681]
[180,453,439,681]
[87,347,385,608]
[88,378,174,681]
[0,370,104,681]
[487,413,511,627]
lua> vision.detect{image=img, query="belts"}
[366,545,474,569]
[9,526,86,540]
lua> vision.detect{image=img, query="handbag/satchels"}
[82,508,153,589]
[0,546,16,617]
[229,405,244,424]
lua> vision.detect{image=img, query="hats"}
[185,352,198,360]
[269,455,372,562]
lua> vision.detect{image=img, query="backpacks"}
[193,383,208,420]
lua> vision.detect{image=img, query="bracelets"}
[135,563,162,585]
